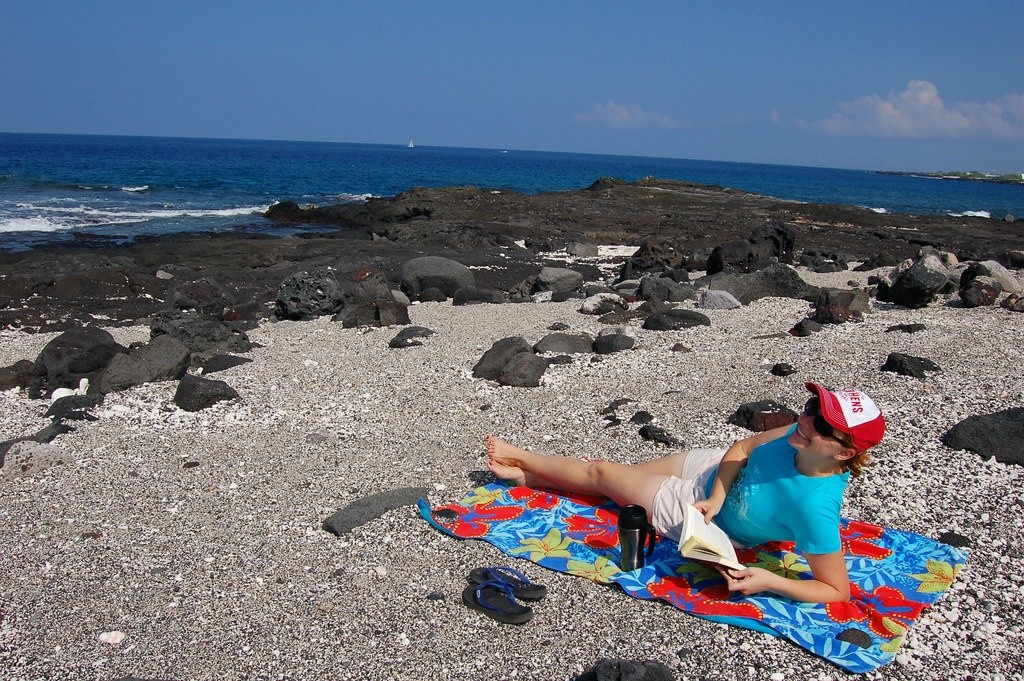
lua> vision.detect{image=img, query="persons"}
[484,382,885,603]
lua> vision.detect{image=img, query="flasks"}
[617,503,656,571]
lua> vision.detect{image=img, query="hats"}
[805,382,885,451]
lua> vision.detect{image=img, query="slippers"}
[462,579,533,623]
[470,567,547,600]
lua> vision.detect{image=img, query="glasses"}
[805,397,849,448]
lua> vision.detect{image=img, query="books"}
[678,503,747,570]
